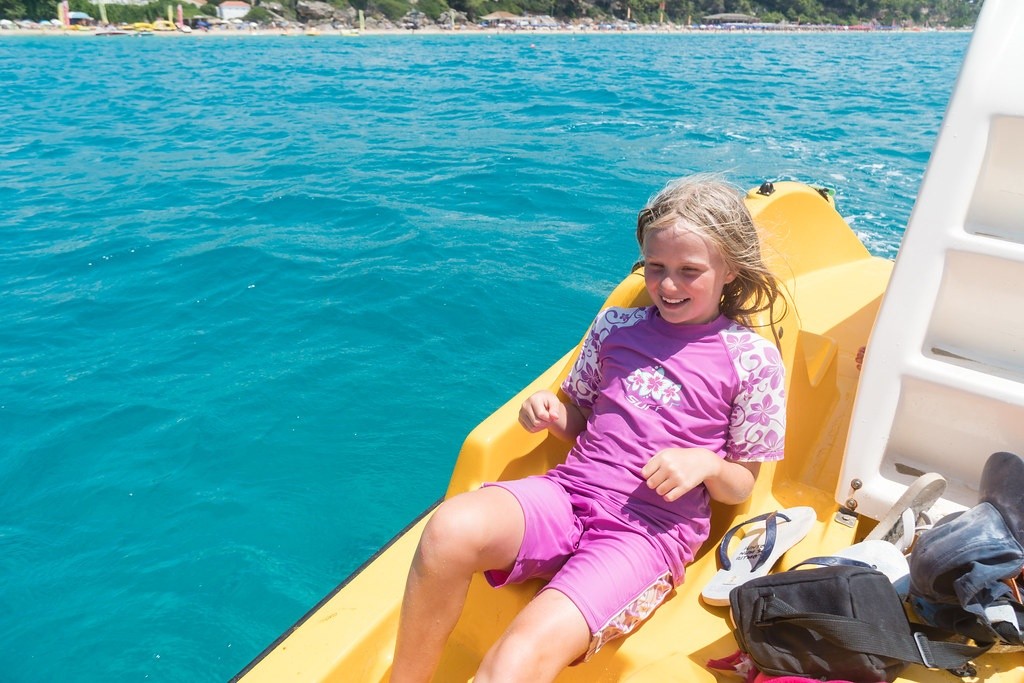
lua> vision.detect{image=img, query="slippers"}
[867,472,947,552]
[787,537,910,606]
[705,503,817,607]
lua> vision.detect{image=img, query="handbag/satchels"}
[728,567,914,683]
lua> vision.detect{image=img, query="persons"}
[390,172,790,683]
